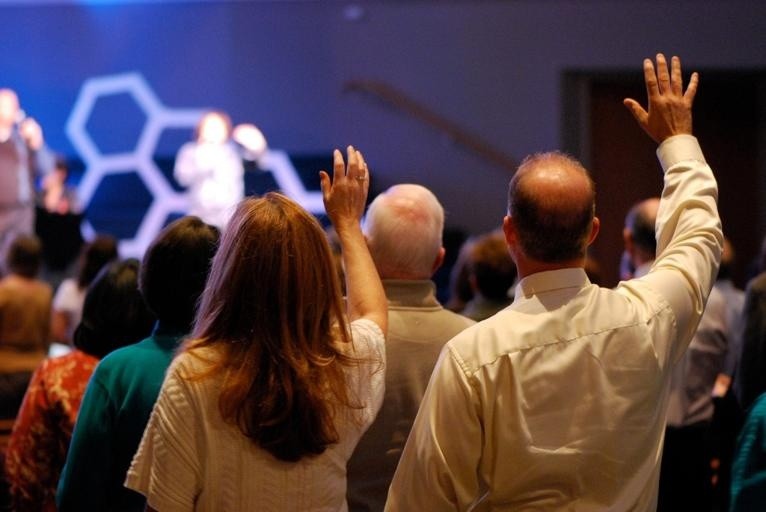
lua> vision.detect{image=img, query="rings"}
[356,176,365,181]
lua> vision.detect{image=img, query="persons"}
[1,89,223,511]
[122,145,389,511]
[383,51,766,511]
[174,112,268,231]
[324,185,518,512]
